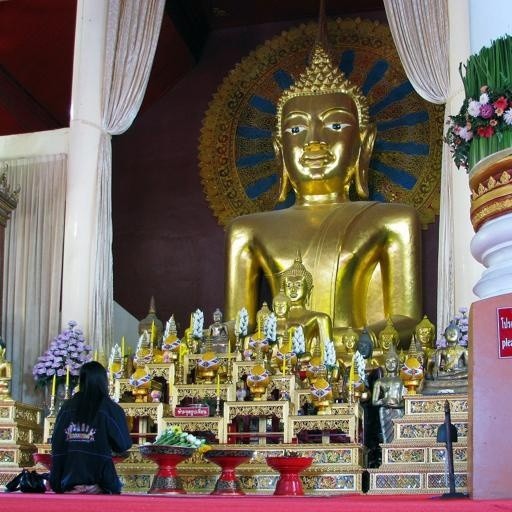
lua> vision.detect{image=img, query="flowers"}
[193,307,366,385]
[31,318,93,393]
[435,305,469,351]
[439,81,511,175]
[154,424,209,447]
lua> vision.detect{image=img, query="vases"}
[137,445,198,494]
[202,449,256,495]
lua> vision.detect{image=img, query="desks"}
[1,491,512,511]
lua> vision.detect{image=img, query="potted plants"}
[264,450,315,496]
[458,31,512,233]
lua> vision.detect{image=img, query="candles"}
[52,313,263,403]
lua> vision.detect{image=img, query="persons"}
[228,42,421,335]
[138,248,467,441]
[49,360,133,495]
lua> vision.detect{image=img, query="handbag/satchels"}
[4,470,52,494]
